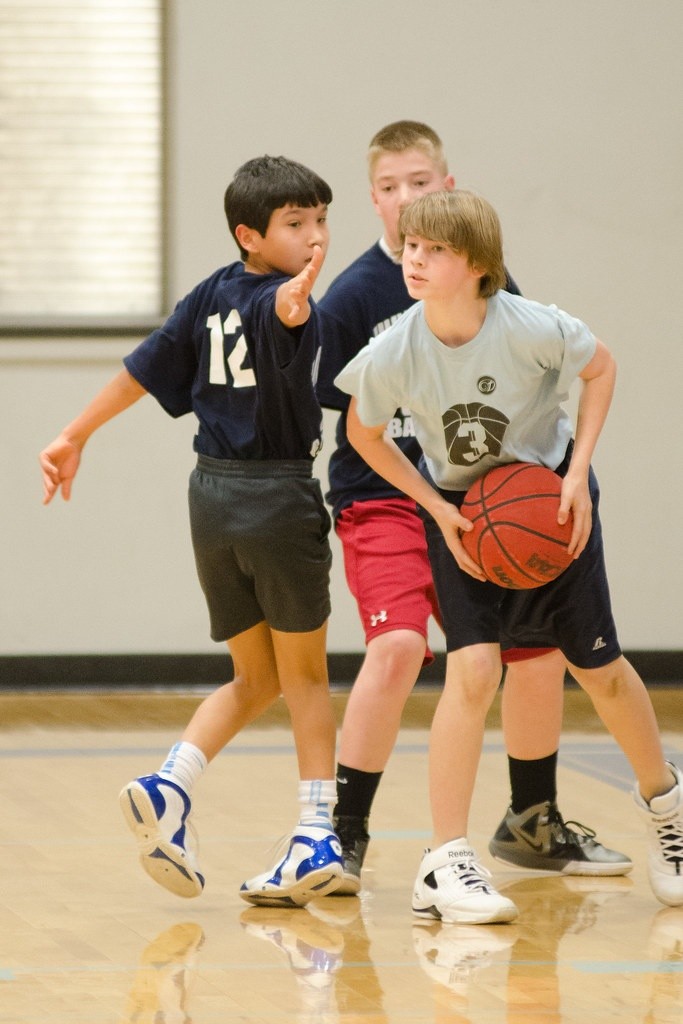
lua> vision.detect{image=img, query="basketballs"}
[456,463,578,591]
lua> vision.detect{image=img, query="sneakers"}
[632,758,683,906]
[414,926,515,986]
[119,771,205,898]
[329,815,370,895]
[117,922,205,1024]
[488,800,633,876]
[411,836,519,924]
[239,908,343,979]
[239,822,345,908]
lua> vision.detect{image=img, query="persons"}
[315,120,634,895]
[334,189,683,926]
[123,874,683,1024]
[37,154,345,907]
